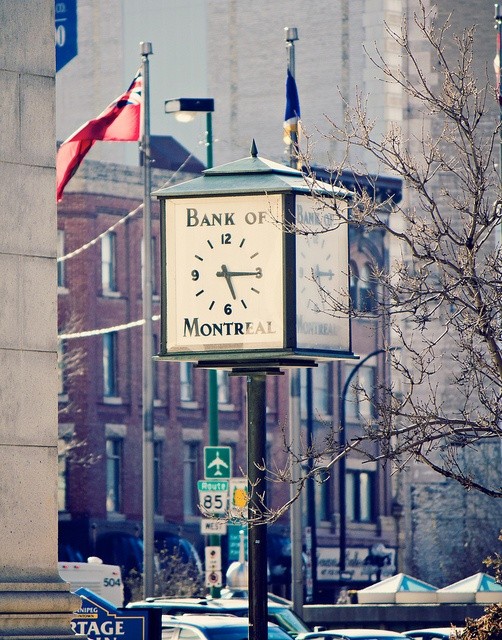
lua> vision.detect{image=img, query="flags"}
[283,67,302,160]
[54,67,140,197]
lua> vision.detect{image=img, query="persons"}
[336,586,351,604]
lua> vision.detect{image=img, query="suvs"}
[125,595,315,639]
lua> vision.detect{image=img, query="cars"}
[295,628,411,640]
[400,627,465,640]
[160,614,295,640]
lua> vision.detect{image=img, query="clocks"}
[161,193,283,358]
[293,190,351,353]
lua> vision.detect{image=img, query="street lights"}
[164,97,224,596]
